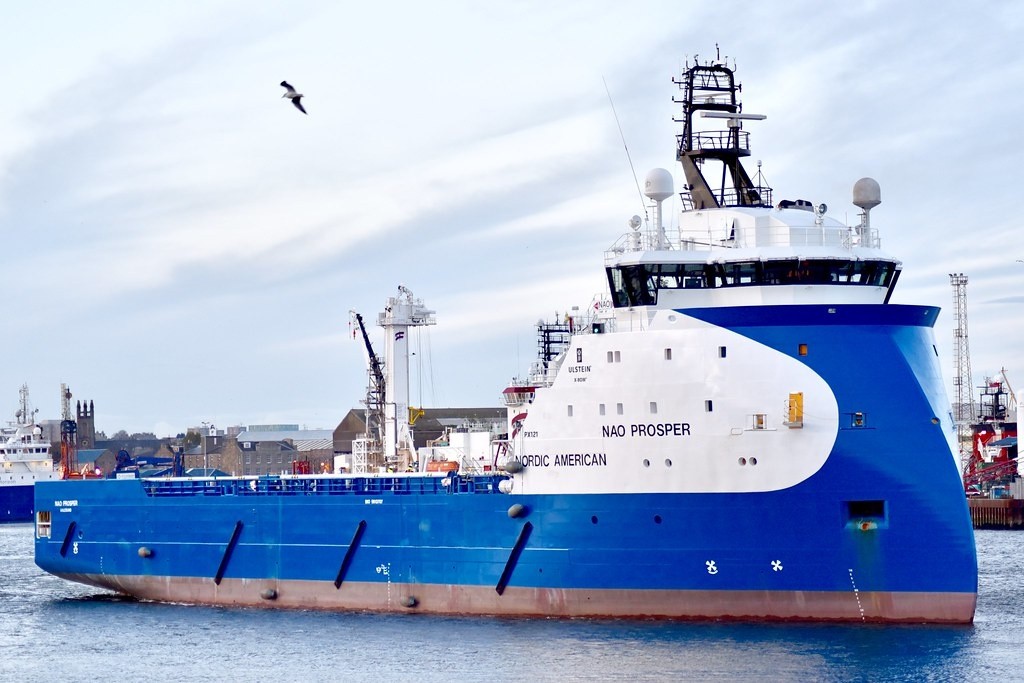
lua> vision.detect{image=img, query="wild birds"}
[279,80,308,115]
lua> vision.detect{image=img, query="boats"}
[0,382,61,525]
[34,41,977,621]
